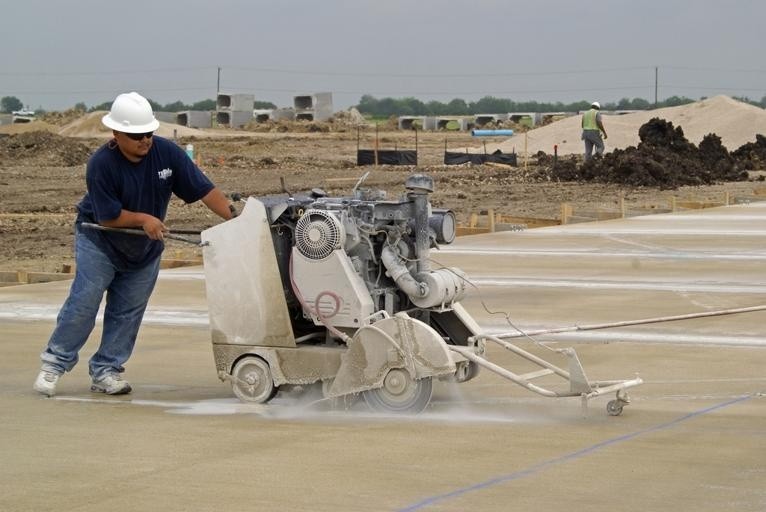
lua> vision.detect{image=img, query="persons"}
[32,90,236,397]
[579,102,607,160]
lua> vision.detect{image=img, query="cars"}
[11,109,38,125]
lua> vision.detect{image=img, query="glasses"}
[124,132,153,141]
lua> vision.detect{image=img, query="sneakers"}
[89,373,132,396]
[33,367,62,398]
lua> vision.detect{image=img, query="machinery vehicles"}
[77,171,645,423]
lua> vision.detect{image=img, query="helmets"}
[591,101,600,108]
[101,92,161,135]
